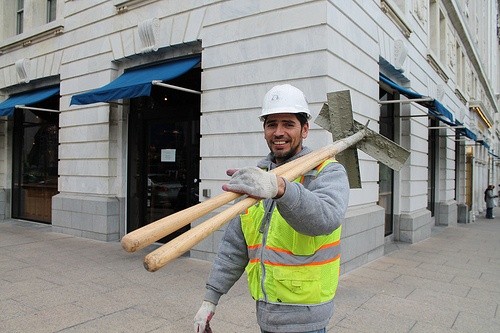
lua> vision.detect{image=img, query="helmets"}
[258,84,313,121]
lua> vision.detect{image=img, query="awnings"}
[69,55,201,106]
[0,88,60,116]
[379,75,453,123]
[466,139,489,148]
[428,118,477,141]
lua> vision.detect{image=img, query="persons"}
[484,184,500,219]
[194,83,350,332]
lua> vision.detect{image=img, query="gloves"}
[223,167,278,198]
[193,301,216,333]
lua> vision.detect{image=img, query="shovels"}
[142,100,413,274]
[120,88,363,254]
